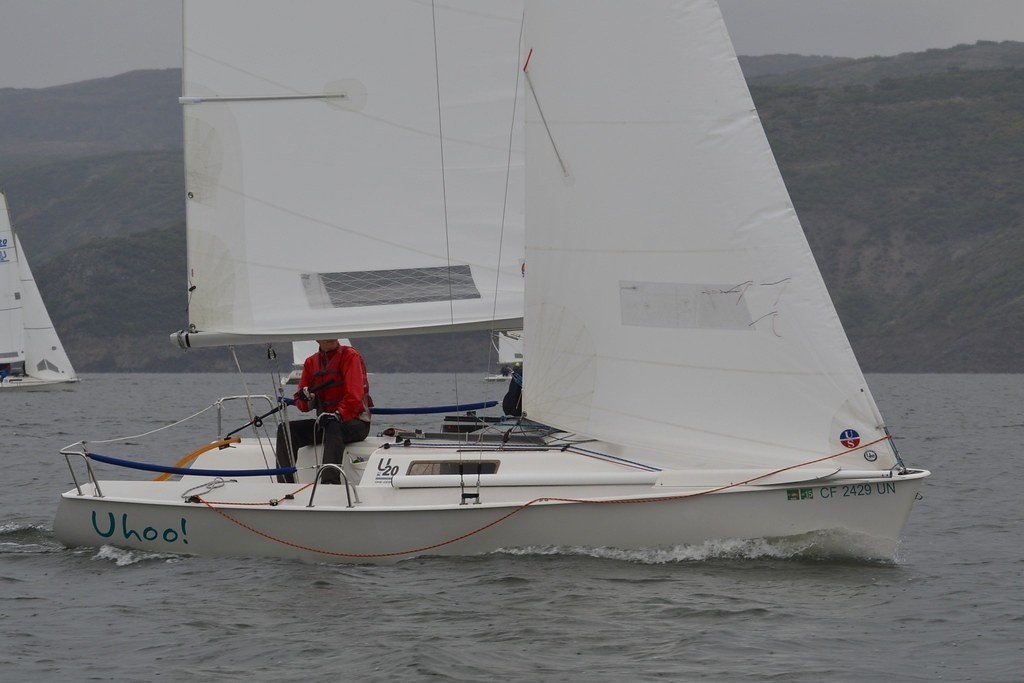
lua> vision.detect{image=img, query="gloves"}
[318,410,341,429]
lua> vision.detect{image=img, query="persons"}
[499,360,525,418]
[271,336,377,484]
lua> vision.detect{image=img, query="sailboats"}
[484,331,522,381]
[283,338,351,385]
[53,3,931,560]
[1,190,82,389]
